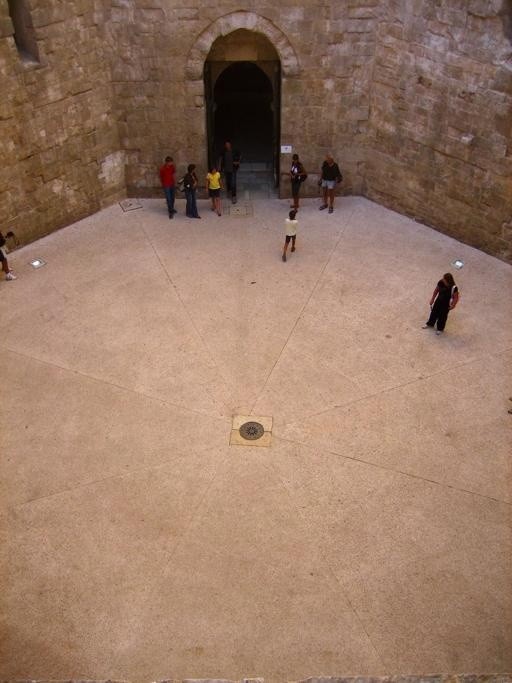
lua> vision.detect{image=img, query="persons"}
[218,141,243,204]
[0,231,16,280]
[422,273,458,335]
[183,163,202,219]
[288,153,308,211]
[205,167,222,217]
[282,210,298,261]
[318,152,343,213]
[159,156,177,220]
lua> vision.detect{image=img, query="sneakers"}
[212,206,221,216]
[169,209,176,218]
[319,204,333,213]
[283,247,295,261]
[187,214,200,218]
[422,325,441,335]
[290,204,298,210]
